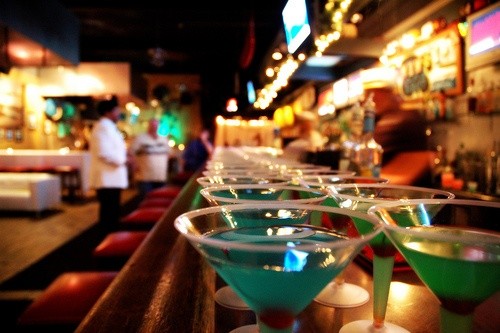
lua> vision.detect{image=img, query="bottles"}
[349,101,383,178]
[456,142,473,190]
[430,146,447,187]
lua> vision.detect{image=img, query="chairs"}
[58,166,87,203]
[11,185,189,333]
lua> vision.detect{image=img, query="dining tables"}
[0,149,91,200]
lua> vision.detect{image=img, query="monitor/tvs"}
[281,0,312,55]
[465,2,500,71]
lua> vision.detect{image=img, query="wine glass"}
[368,200,500,332]
[173,144,388,332]
[325,184,455,332]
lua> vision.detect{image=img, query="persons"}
[87,94,128,223]
[199,129,215,158]
[282,108,327,163]
[363,78,434,186]
[128,116,171,203]
[181,126,210,173]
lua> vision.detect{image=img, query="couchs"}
[0,173,60,216]
